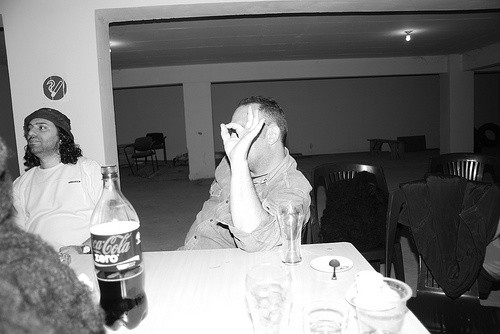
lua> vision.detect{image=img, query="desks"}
[57,241,430,334]
[367,139,404,159]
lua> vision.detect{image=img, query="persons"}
[174,95,312,254]
[9,108,110,248]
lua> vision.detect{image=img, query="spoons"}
[329,259,340,280]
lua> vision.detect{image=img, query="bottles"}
[88,163,148,332]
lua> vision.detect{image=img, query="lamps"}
[405,31,413,41]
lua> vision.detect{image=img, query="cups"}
[246,262,293,334]
[277,199,303,265]
[304,272,414,334]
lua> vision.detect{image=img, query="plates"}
[310,255,354,273]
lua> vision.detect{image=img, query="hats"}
[24,107,75,142]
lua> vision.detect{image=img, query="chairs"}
[118,133,167,175]
[303,152,500,334]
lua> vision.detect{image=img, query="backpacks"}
[320,171,388,253]
